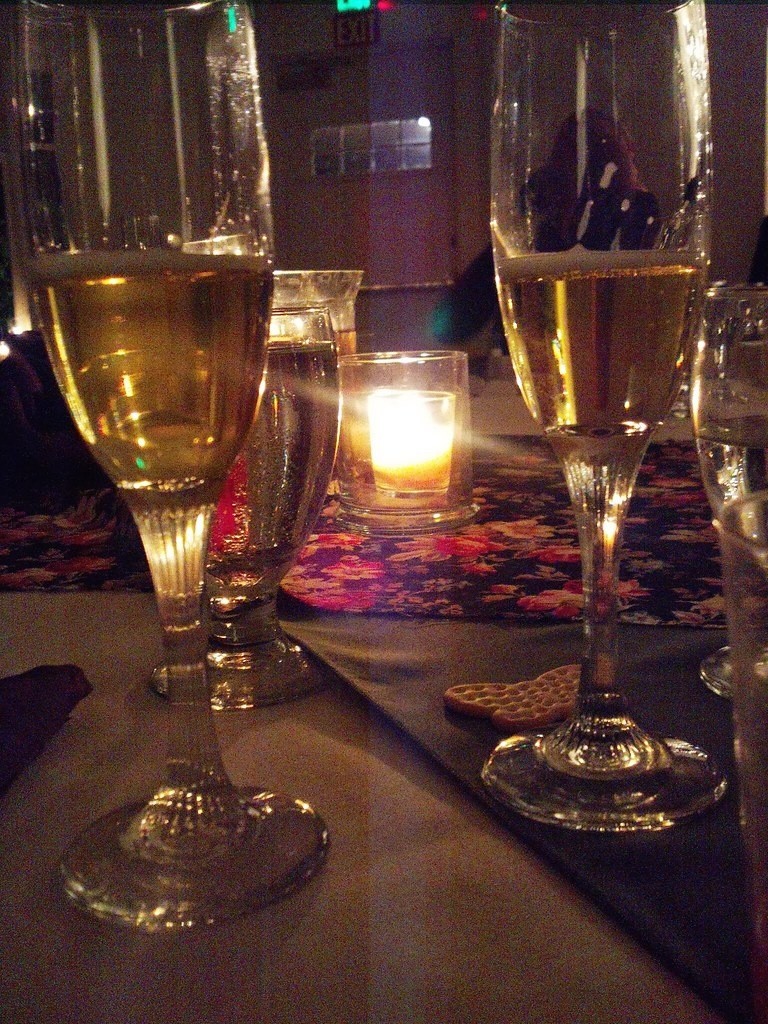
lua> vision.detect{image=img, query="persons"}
[0,325,112,511]
[429,103,662,384]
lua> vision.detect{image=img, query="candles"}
[371,390,449,496]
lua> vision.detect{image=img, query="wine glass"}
[691,286,768,699]
[151,308,339,712]
[477,0,728,833]
[0,0,332,933]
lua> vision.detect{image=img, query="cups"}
[335,350,481,535]
[716,490,768,1024]
[274,271,363,355]
